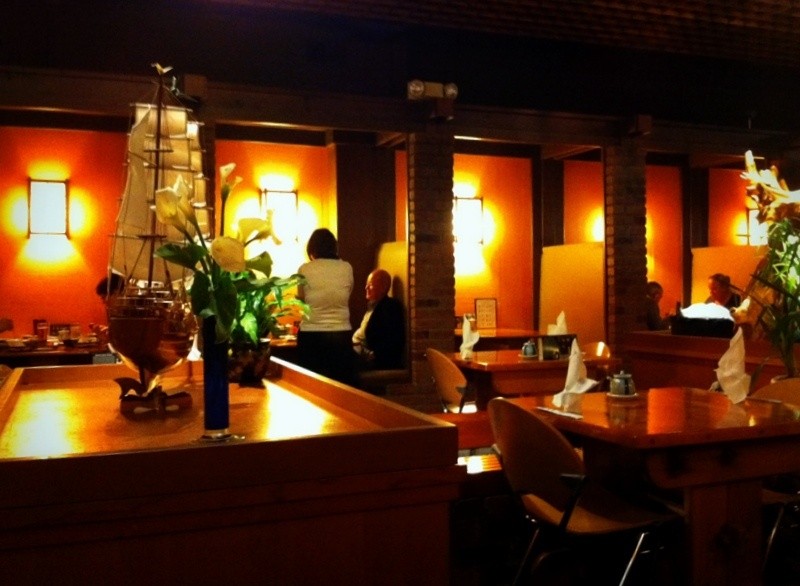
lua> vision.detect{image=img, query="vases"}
[228,339,270,382]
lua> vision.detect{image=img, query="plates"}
[607,393,638,398]
[518,354,539,359]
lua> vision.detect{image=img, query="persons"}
[296,229,358,388]
[351,269,408,370]
[705,273,740,312]
[647,282,672,330]
[88,274,126,342]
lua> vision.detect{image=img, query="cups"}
[70,323,81,340]
[608,370,636,395]
[37,322,48,341]
[522,341,536,355]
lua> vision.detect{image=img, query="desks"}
[273,328,800,586]
[0,341,110,369]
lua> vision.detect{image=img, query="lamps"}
[25,175,71,240]
[452,196,484,245]
[407,81,459,104]
[257,187,299,243]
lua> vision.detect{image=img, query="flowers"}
[149,159,313,350]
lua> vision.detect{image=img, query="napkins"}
[459,316,480,360]
[714,326,751,404]
[552,338,602,414]
[547,311,567,335]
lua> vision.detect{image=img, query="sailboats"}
[107,63,197,394]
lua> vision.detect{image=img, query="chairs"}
[488,398,679,586]
[422,346,478,414]
[749,377,800,576]
